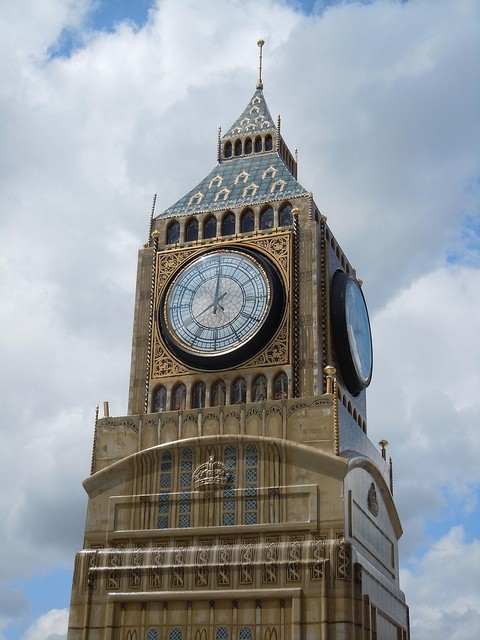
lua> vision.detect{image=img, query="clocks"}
[331,269,374,395]
[157,244,285,371]
